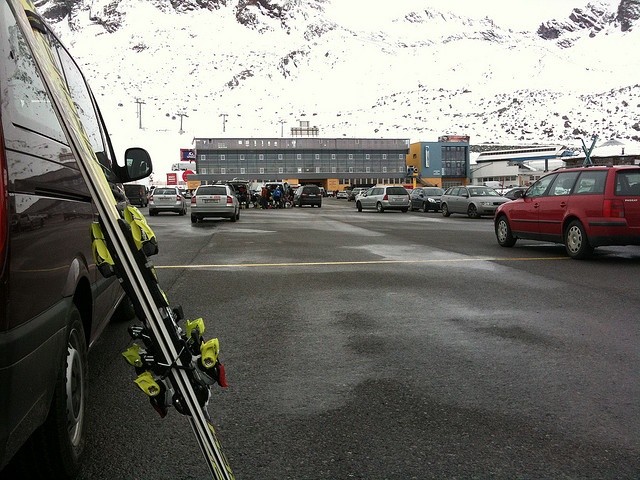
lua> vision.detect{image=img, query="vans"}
[124,185,147,207]
[0,0,153,477]
[264,181,291,208]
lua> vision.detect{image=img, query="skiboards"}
[4,0,237,478]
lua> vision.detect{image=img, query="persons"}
[260,184,284,209]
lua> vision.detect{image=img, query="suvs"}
[348,192,357,201]
[226,180,251,209]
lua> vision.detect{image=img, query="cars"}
[493,164,640,259]
[292,185,322,208]
[147,186,188,217]
[503,186,530,200]
[439,185,512,219]
[354,184,411,213]
[410,186,446,213]
[190,184,240,222]
[336,191,348,199]
